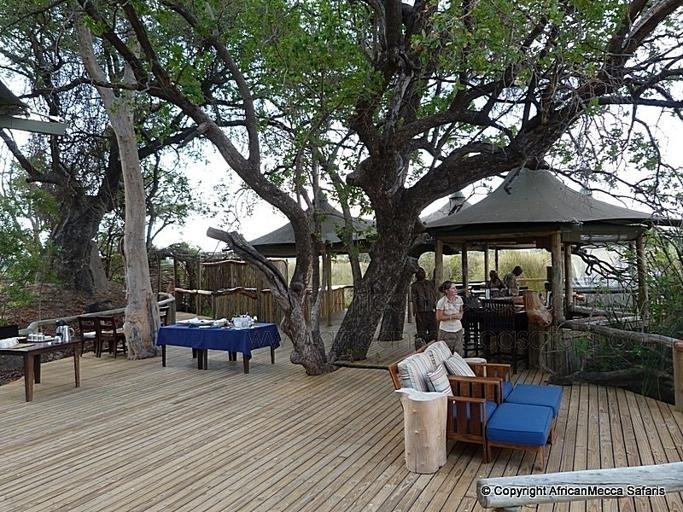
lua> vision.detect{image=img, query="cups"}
[27,332,45,341]
[0,337,18,349]
[54,333,63,344]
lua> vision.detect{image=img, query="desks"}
[155,319,281,375]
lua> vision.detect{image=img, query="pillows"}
[425,365,455,405]
[444,352,476,378]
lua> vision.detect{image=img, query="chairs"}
[416,339,564,441]
[76,305,170,358]
[389,350,556,472]
[0,324,41,385]
[460,286,552,374]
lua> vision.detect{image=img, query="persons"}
[435,279,464,357]
[502,265,522,296]
[429,267,435,285]
[410,266,435,350]
[486,270,505,289]
[538,293,545,306]
